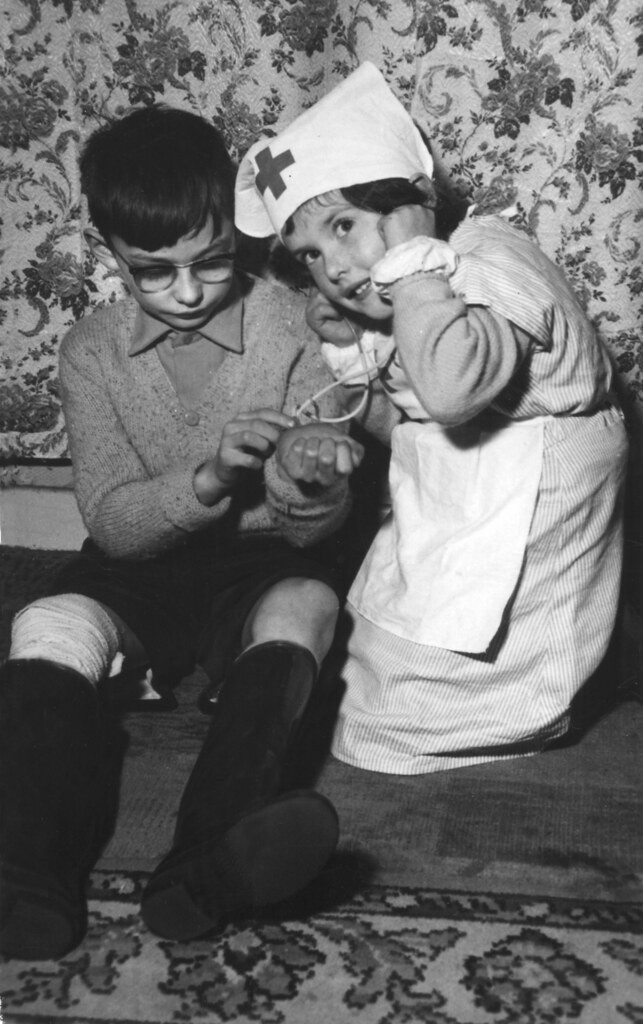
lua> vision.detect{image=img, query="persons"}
[232,61,631,775]
[0,108,363,961]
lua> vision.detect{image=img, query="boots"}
[0,660,101,962]
[139,640,340,943]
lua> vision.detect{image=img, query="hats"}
[235,61,433,243]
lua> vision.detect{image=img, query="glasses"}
[100,229,240,293]
